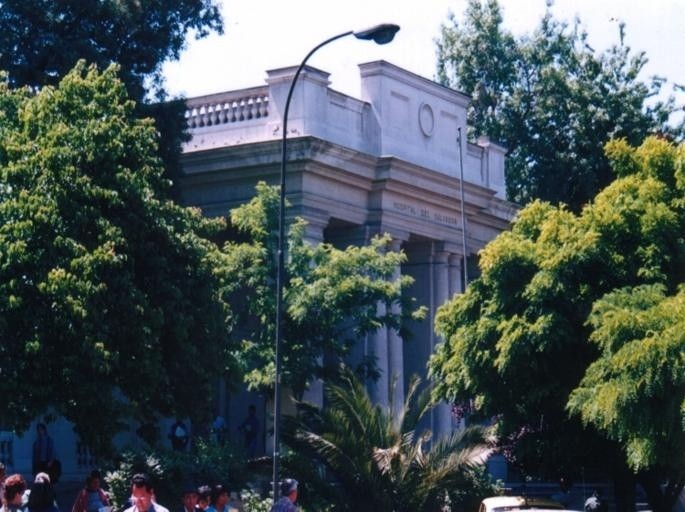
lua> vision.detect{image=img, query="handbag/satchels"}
[46,459,61,484]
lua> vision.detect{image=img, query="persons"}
[584,487,607,512]
[1,405,303,512]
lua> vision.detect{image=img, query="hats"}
[179,484,201,497]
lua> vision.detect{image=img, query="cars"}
[583,488,602,510]
[478,494,568,510]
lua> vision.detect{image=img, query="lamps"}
[468,130,490,147]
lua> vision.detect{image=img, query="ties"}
[36,439,41,464]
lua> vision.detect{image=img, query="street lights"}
[266,23,403,511]
